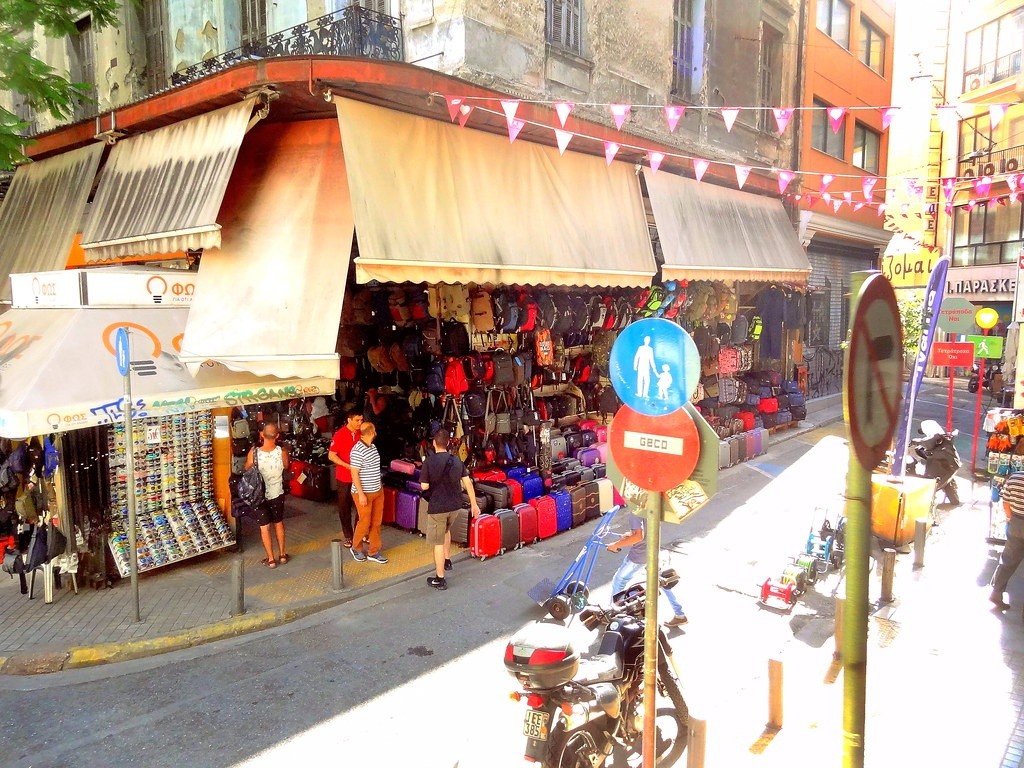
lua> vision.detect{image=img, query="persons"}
[607,512,688,627]
[244,422,289,569]
[418,429,481,590]
[328,407,370,549]
[0,503,15,564]
[989,470,1024,610]
[349,422,388,564]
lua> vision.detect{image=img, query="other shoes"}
[664,615,688,628]
[989,597,1009,609]
[344,538,353,548]
[362,535,370,544]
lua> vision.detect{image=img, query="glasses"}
[107,410,235,576]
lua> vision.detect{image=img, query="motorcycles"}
[504,569,691,768]
[871,419,960,507]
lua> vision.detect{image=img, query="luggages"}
[288,460,323,501]
[450,466,556,562]
[542,419,626,541]
[382,456,430,537]
[718,427,769,469]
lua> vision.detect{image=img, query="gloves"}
[982,408,1024,476]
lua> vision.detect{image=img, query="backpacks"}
[230,447,270,524]
[685,279,763,373]
[337,280,694,397]
[536,385,617,425]
[230,419,250,439]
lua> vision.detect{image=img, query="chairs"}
[979,374,1014,420]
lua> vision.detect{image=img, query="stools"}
[28,555,78,604]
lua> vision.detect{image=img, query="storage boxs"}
[504,622,579,690]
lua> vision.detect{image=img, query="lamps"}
[968,149,983,159]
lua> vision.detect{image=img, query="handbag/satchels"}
[441,392,541,467]
[0,437,67,574]
[422,455,454,502]
[690,372,808,440]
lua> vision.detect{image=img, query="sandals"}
[260,558,277,568]
[278,554,288,565]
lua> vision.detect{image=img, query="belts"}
[61,424,113,554]
[1012,513,1024,520]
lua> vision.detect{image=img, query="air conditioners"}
[963,155,1024,181]
[968,72,989,91]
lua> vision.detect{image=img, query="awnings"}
[331,95,812,287]
[0,91,354,440]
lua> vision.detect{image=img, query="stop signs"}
[611,401,702,493]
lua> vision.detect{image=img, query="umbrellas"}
[1001,321,1019,407]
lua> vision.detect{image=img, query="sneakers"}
[427,575,447,590]
[444,562,452,571]
[367,553,388,564]
[349,546,366,561]
